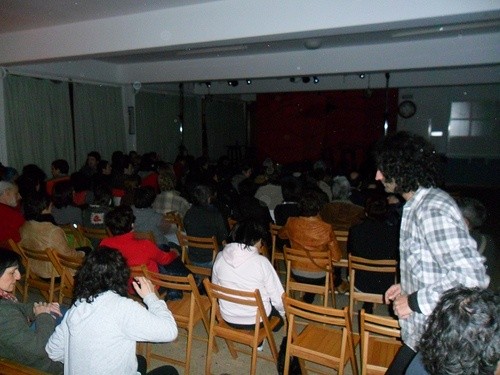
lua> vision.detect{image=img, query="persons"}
[0,148,500,375]
[374,132,492,375]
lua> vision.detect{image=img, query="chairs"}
[280,292,361,375]
[59,223,81,244]
[163,214,185,232]
[348,253,397,339]
[52,248,84,305]
[360,309,403,375]
[329,230,349,295]
[283,244,336,327]
[77,224,112,241]
[0,238,27,295]
[16,242,66,303]
[142,266,220,375]
[203,278,280,375]
[269,222,287,274]
[176,230,219,277]
[227,217,269,257]
